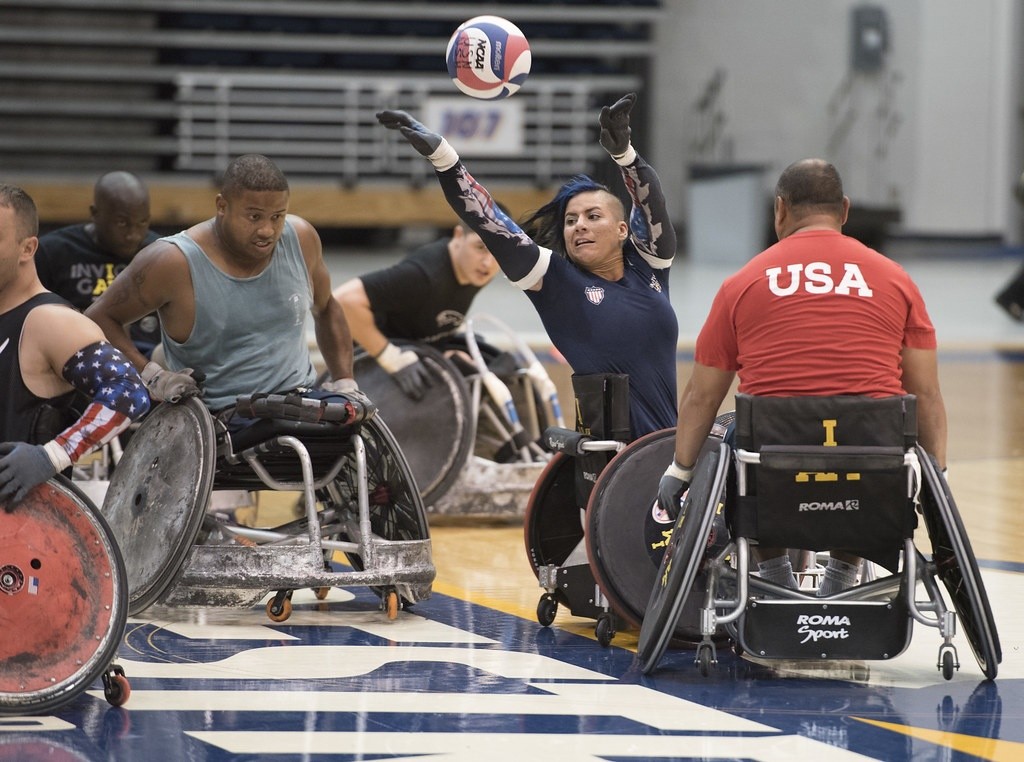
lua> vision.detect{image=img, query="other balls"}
[446,14,533,102]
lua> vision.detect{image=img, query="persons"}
[330,201,542,463]
[656,158,948,599]
[0,184,152,513]
[35,168,165,482]
[82,154,360,491]
[374,90,679,442]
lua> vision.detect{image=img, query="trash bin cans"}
[768,194,903,254]
[679,159,771,265]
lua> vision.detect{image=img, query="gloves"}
[599,93,639,166]
[375,109,460,173]
[657,452,697,520]
[376,341,434,401]
[1,441,73,514]
[139,362,206,404]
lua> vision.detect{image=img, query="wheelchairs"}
[0,470,131,716]
[316,310,566,521]
[522,372,1005,682]
[103,388,435,625]
